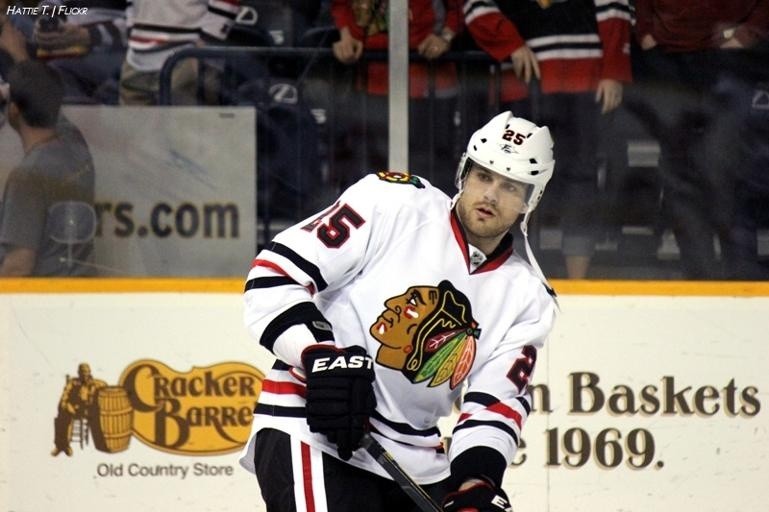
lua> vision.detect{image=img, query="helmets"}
[454,110,555,214]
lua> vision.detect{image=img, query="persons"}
[5,1,768,275]
[238,109,558,512]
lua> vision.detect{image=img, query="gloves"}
[302,344,377,460]
[442,482,513,512]
